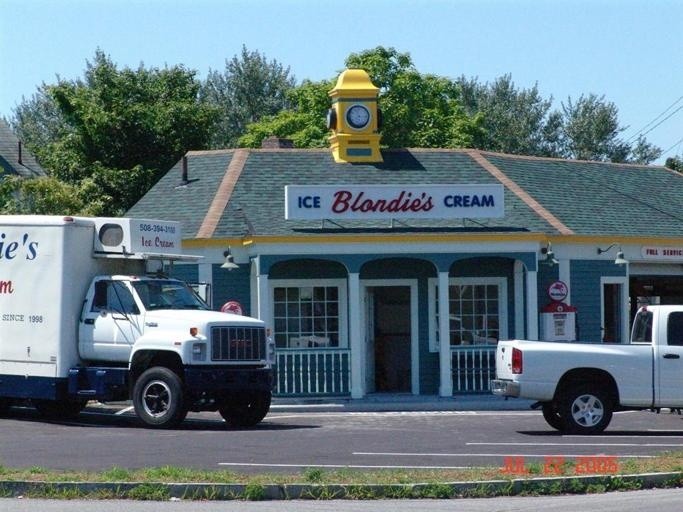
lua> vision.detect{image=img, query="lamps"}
[598,242,629,266]
[542,240,559,268]
[219,243,239,272]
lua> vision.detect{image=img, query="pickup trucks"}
[489,301,682,436]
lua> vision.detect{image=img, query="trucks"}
[0,211,274,427]
